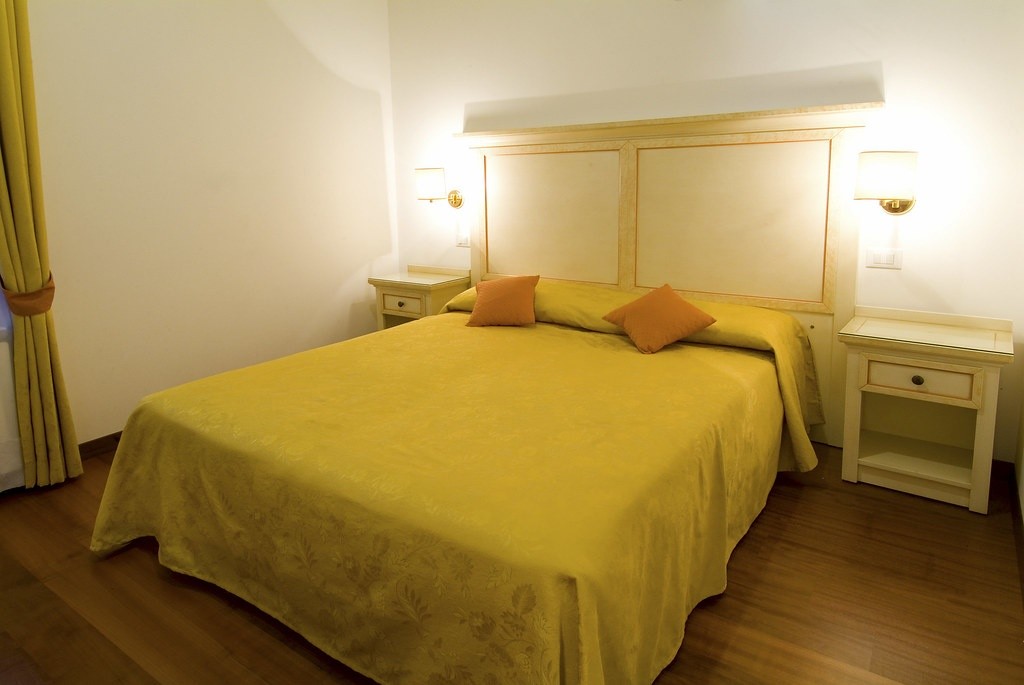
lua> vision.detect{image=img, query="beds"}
[130,273,809,685]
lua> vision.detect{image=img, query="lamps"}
[851,149,918,215]
[411,166,463,208]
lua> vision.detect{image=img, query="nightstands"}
[367,265,472,331]
[837,314,1015,516]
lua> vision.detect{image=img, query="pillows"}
[465,275,540,327]
[602,284,716,355]
